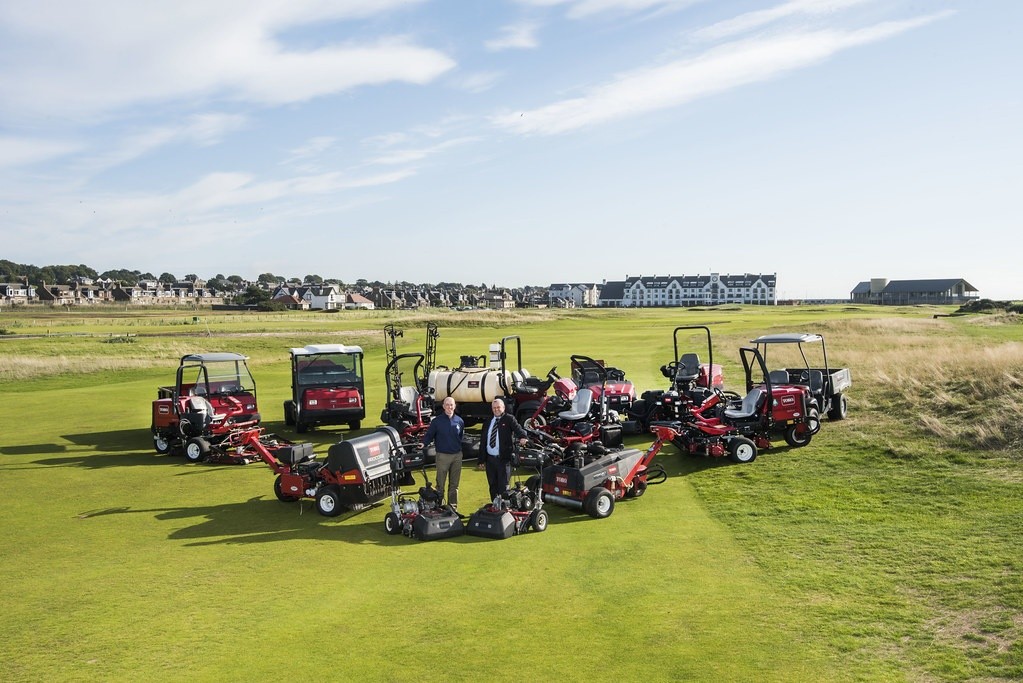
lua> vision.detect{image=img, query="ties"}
[490,418,499,449]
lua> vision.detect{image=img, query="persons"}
[417,396,464,509]
[476,399,528,502]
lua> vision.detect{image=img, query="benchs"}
[300,364,350,382]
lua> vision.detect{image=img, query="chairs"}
[559,389,593,422]
[581,372,599,384]
[802,370,824,394]
[186,387,208,396]
[219,384,238,393]
[724,389,765,419]
[674,353,700,382]
[512,370,539,393]
[519,368,542,386]
[769,370,790,384]
[604,371,619,380]
[400,385,432,416]
